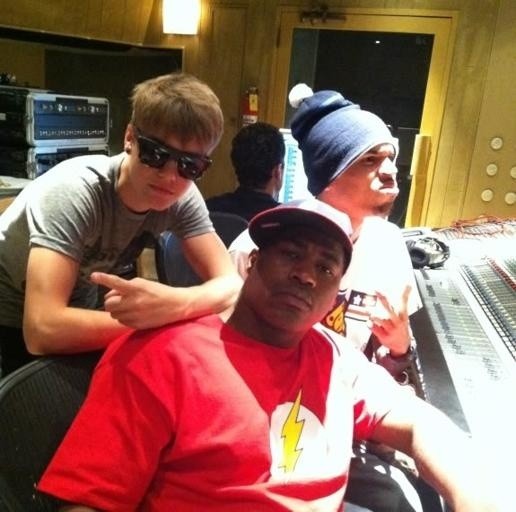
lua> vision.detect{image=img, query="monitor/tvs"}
[273,128,399,204]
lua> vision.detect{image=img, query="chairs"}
[0,351,105,512]
[155,212,248,286]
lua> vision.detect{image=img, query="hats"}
[288,83,399,199]
[248,198,359,278]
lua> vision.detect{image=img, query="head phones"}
[406,236,449,269]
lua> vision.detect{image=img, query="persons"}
[0,72,245,387]
[35,197,515,512]
[229,82,443,512]
[205,121,286,221]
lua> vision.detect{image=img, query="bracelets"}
[388,334,417,362]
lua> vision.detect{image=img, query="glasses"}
[134,128,213,182]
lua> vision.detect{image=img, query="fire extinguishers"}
[239,85,259,130]
